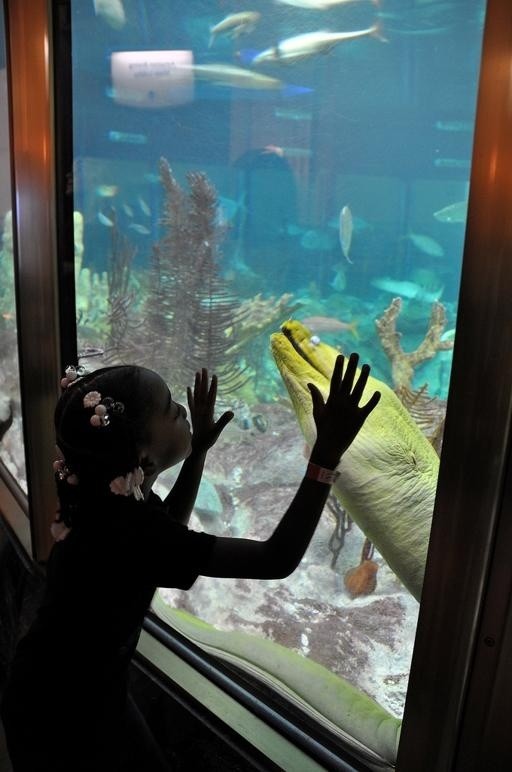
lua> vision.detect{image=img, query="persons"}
[2,353,380,771]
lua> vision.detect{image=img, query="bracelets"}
[304,458,342,485]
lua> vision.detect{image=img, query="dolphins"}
[269,317,442,606]
